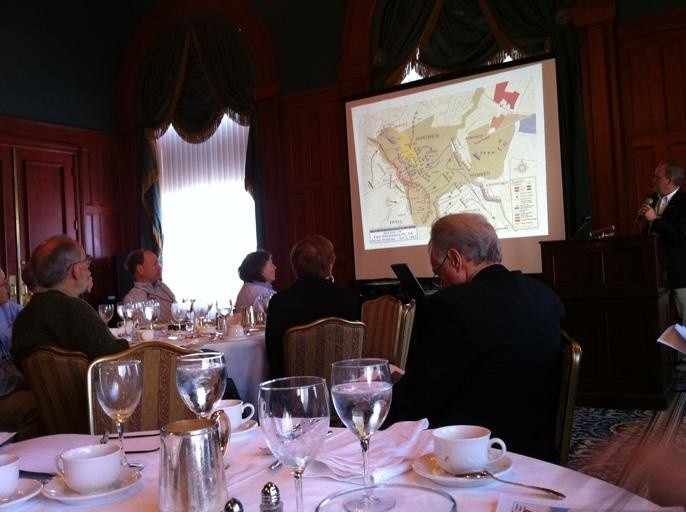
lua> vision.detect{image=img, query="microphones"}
[634,195,656,223]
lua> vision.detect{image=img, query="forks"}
[248,422,303,455]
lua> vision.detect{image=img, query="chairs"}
[282,293,416,428]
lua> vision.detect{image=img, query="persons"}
[124,249,176,322]
[235,250,277,312]
[265,234,352,377]
[13,234,128,375]
[22,260,43,306]
[1,265,39,442]
[634,161,686,380]
[381,212,566,463]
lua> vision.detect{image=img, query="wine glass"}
[329,358,397,510]
[258,374,327,511]
[94,298,234,348]
[94,359,145,470]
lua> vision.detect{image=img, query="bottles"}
[258,482,285,512]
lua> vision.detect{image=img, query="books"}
[655,324,686,356]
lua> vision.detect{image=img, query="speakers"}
[103,255,134,301]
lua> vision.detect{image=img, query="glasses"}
[65,254,93,273]
[431,255,448,288]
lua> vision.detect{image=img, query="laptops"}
[390,263,439,297]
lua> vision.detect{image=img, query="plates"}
[413,452,514,486]
[0,475,42,508]
[44,474,149,505]
[192,419,259,438]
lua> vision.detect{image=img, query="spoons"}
[453,469,568,500]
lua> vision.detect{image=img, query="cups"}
[174,351,227,414]
[0,453,22,502]
[430,423,505,472]
[208,399,259,424]
[54,444,126,495]
[241,305,254,331]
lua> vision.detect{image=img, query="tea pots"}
[155,410,233,512]
